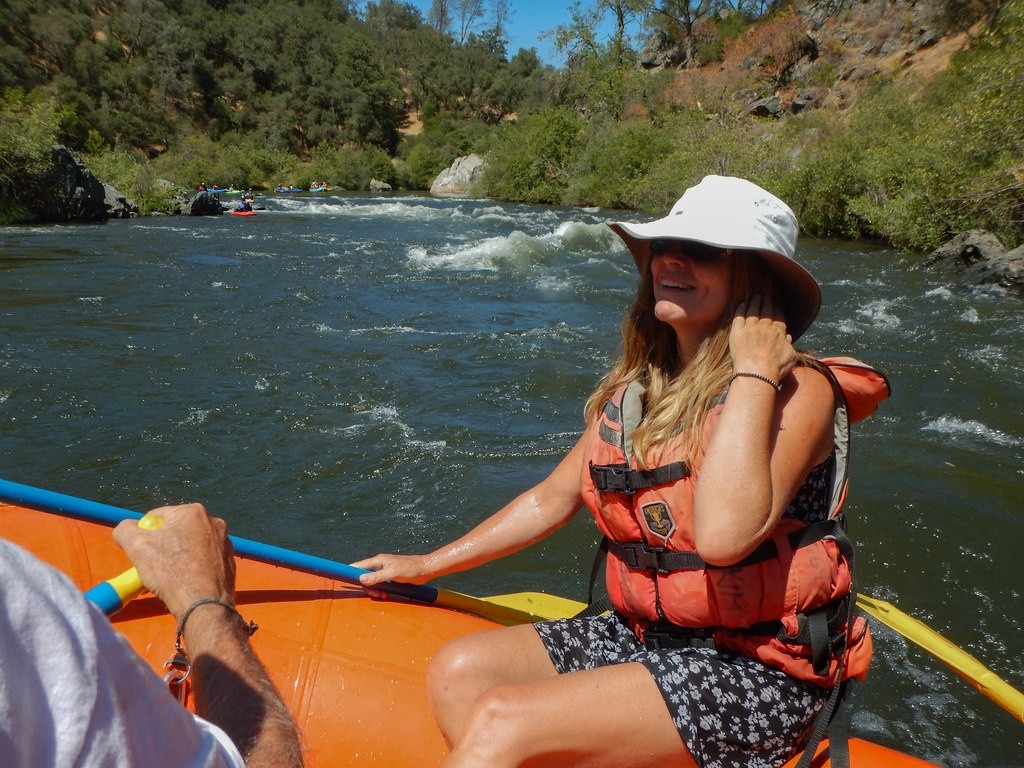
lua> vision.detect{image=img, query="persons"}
[322,181,327,188]
[311,181,318,189]
[249,188,252,195]
[213,183,220,190]
[196,182,207,190]
[348,174,892,768]
[229,183,235,192]
[235,193,253,211]
[288,183,293,190]
[276,184,282,190]
[0,502,305,768]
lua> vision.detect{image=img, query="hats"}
[605,174,822,346]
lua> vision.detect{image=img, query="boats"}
[226,190,245,195]
[309,188,323,192]
[322,188,333,191]
[207,189,228,192]
[231,211,257,216]
[274,189,301,192]
[245,198,254,202]
[0,480,1024,768]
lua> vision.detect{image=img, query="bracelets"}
[729,371,782,392]
[165,597,259,685]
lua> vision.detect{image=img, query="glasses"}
[649,238,731,265]
